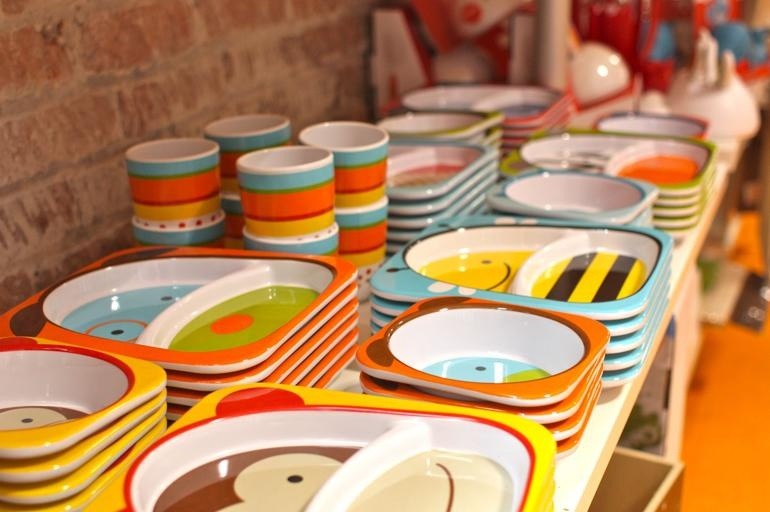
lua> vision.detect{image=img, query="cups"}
[125,137,225,246]
[204,112,293,243]
[237,142,340,263]
[297,121,390,271]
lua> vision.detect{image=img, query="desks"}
[0,79,766,512]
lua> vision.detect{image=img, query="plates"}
[377,81,718,261]
[0,242,362,423]
[0,331,168,512]
[82,382,557,512]
[367,211,672,384]
[354,295,610,456]
